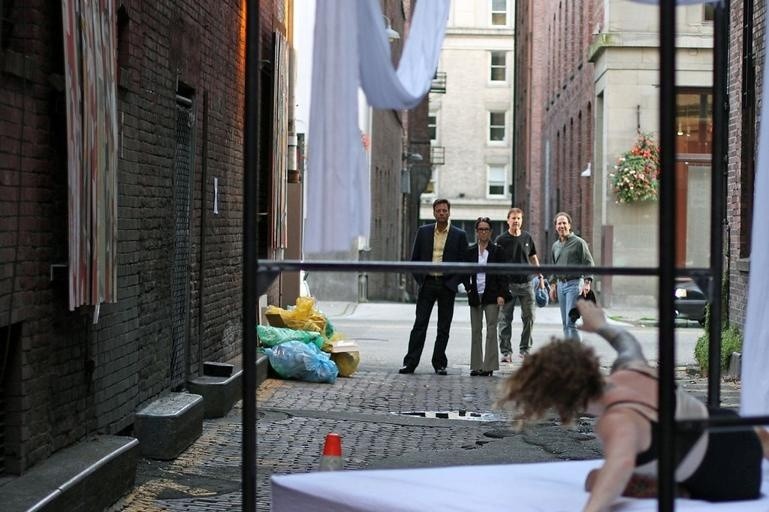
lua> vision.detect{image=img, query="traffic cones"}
[316,431,344,472]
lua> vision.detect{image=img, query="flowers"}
[609,127,663,208]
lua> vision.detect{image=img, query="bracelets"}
[537,275,545,280]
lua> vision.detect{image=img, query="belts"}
[561,279,566,283]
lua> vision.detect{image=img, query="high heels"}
[470,369,482,376]
[479,370,493,376]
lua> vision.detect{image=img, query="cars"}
[675,278,707,328]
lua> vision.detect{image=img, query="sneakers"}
[518,352,529,363]
[500,353,512,364]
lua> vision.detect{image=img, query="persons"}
[461,215,509,377]
[492,293,769,512]
[397,198,469,375]
[492,206,548,363]
[546,208,599,350]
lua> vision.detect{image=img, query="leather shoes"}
[399,366,414,374]
[437,367,447,375]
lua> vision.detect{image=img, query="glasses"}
[477,217,489,222]
[478,228,491,233]
